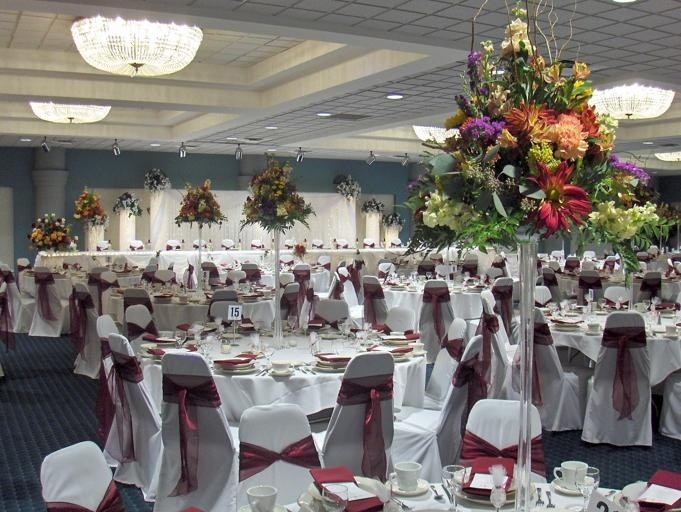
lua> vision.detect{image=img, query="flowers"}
[400,0,681,288]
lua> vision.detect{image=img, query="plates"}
[615,480,648,512]
[552,477,595,495]
[111,282,278,304]
[130,317,430,379]
[308,475,391,512]
[455,466,516,504]
[539,296,680,339]
[386,478,430,496]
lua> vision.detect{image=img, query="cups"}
[323,485,348,511]
[553,459,590,491]
[575,467,601,512]
[390,462,423,490]
[247,486,278,512]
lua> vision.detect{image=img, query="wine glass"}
[380,271,488,294]
[490,485,507,512]
[444,465,466,511]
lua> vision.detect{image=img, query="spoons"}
[431,485,444,499]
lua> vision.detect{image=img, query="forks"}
[536,487,545,506]
[391,496,411,511]
[547,491,555,508]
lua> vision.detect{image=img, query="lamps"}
[654,152,681,161]
[587,83,676,120]
[69,15,204,77]
[31,102,111,123]
[412,125,460,143]
